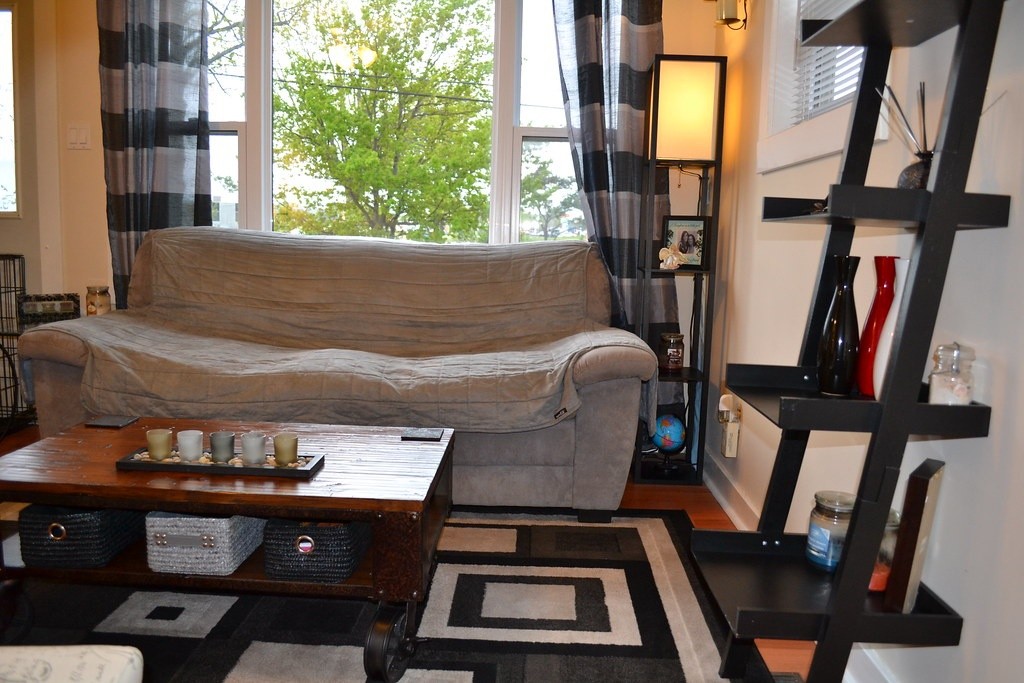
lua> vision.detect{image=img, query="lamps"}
[643,54,728,166]
[715,0,747,31]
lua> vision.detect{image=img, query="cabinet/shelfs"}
[690,1,1010,683]
[634,165,721,487]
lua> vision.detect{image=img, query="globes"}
[650,414,690,475]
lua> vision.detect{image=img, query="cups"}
[209,432,235,463]
[147,429,172,460]
[273,433,298,466]
[240,432,266,464]
[177,430,203,461]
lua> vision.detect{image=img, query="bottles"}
[815,255,976,406]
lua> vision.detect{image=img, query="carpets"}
[0,505,778,683]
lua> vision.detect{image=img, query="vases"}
[817,256,910,401]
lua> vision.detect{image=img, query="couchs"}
[17,226,658,524]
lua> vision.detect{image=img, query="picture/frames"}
[663,215,713,271]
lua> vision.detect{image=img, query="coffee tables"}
[0,416,455,683]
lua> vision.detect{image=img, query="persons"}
[677,231,696,255]
